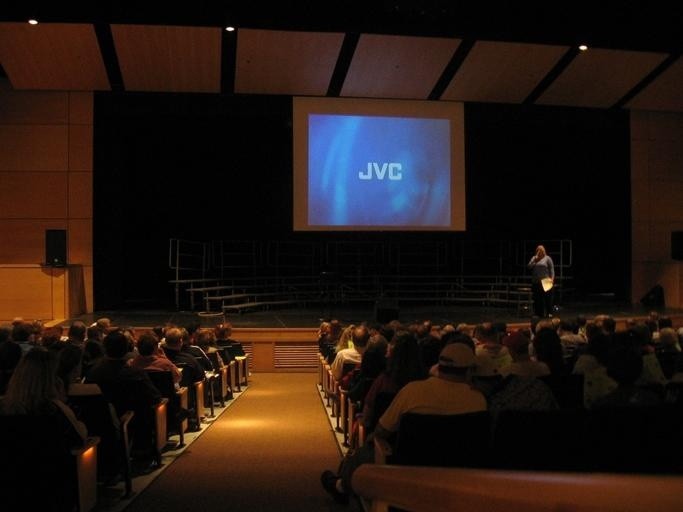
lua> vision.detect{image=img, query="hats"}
[438,344,513,376]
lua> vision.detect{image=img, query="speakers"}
[640,285,665,308]
[374,301,399,323]
[670,230,683,260]
[46,230,67,268]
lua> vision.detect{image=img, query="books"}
[541,277,554,292]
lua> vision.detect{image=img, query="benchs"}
[170,276,576,316]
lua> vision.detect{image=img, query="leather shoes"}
[321,470,344,506]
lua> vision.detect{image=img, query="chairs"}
[0,343,250,512]
[317,351,683,512]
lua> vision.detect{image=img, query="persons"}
[321,314,683,508]
[526,245,555,317]
[1,318,245,512]
